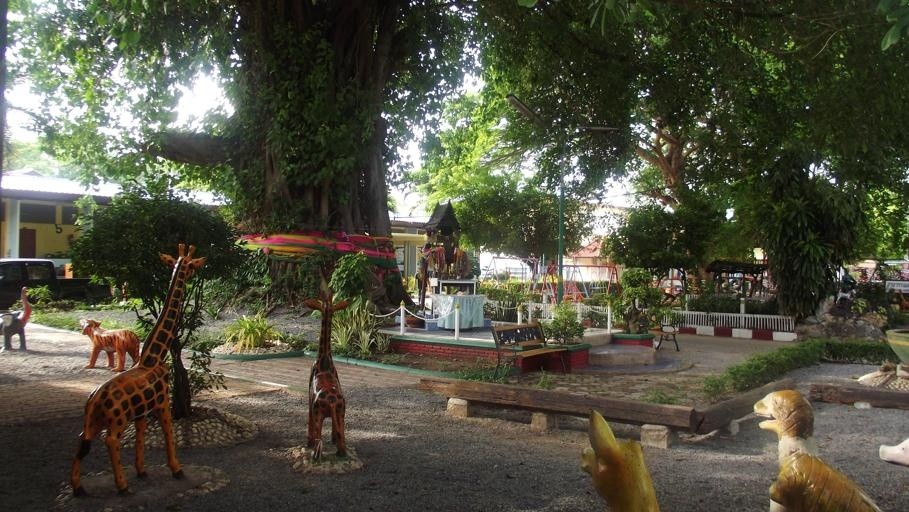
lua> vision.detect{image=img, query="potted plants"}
[405,306,424,328]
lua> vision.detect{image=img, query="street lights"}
[506,93,619,339]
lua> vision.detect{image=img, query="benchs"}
[490,320,567,384]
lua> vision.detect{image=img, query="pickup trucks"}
[0,258,112,308]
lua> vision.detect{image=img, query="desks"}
[429,294,487,333]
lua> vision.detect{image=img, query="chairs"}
[899,289,909,312]
[655,314,680,351]
